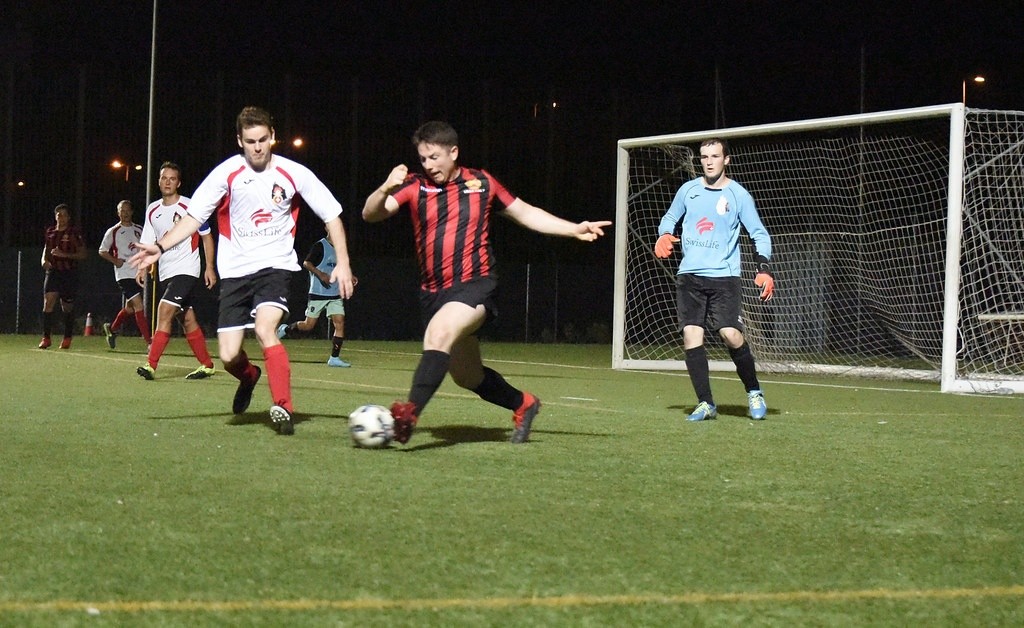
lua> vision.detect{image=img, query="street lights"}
[112,161,129,181]
[962,76,985,104]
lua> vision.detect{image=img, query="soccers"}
[347,404,396,449]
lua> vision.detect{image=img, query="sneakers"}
[389,401,417,443]
[328,355,352,368]
[186,362,216,380]
[277,323,288,339]
[104,322,118,349]
[38,338,52,348]
[270,406,294,435]
[59,337,73,349]
[136,363,156,380]
[233,365,262,413]
[512,391,542,444]
[686,401,717,421]
[747,388,767,419]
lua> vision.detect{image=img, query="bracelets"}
[156,243,164,255]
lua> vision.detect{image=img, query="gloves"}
[655,233,680,258]
[754,271,774,301]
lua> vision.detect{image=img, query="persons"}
[127,107,353,435]
[655,137,775,423]
[135,162,217,381]
[276,226,359,368]
[99,200,152,354]
[362,122,612,445]
[38,203,86,350]
